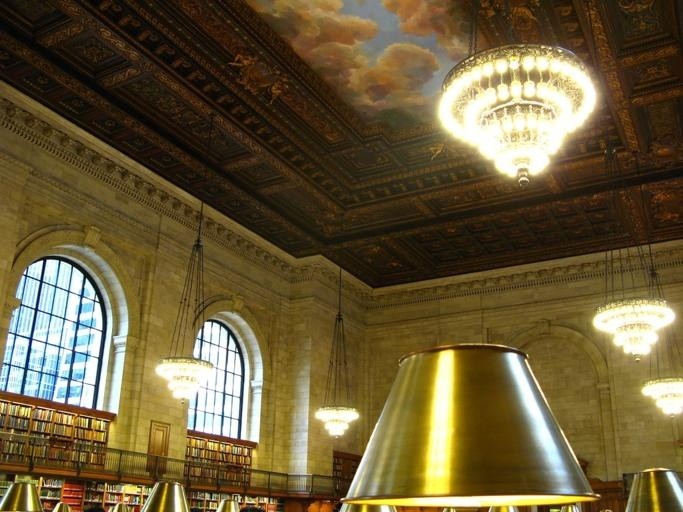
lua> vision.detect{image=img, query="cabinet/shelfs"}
[0,390,116,472]
[188,429,258,486]
[0,466,286,512]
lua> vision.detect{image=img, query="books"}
[184,438,251,487]
[0,402,108,470]
[185,491,279,512]
[332,457,358,497]
[0,474,154,511]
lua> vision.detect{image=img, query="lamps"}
[312,211,364,438]
[622,465,682,511]
[586,146,682,420]
[435,0,598,188]
[339,344,602,512]
[154,91,216,407]
